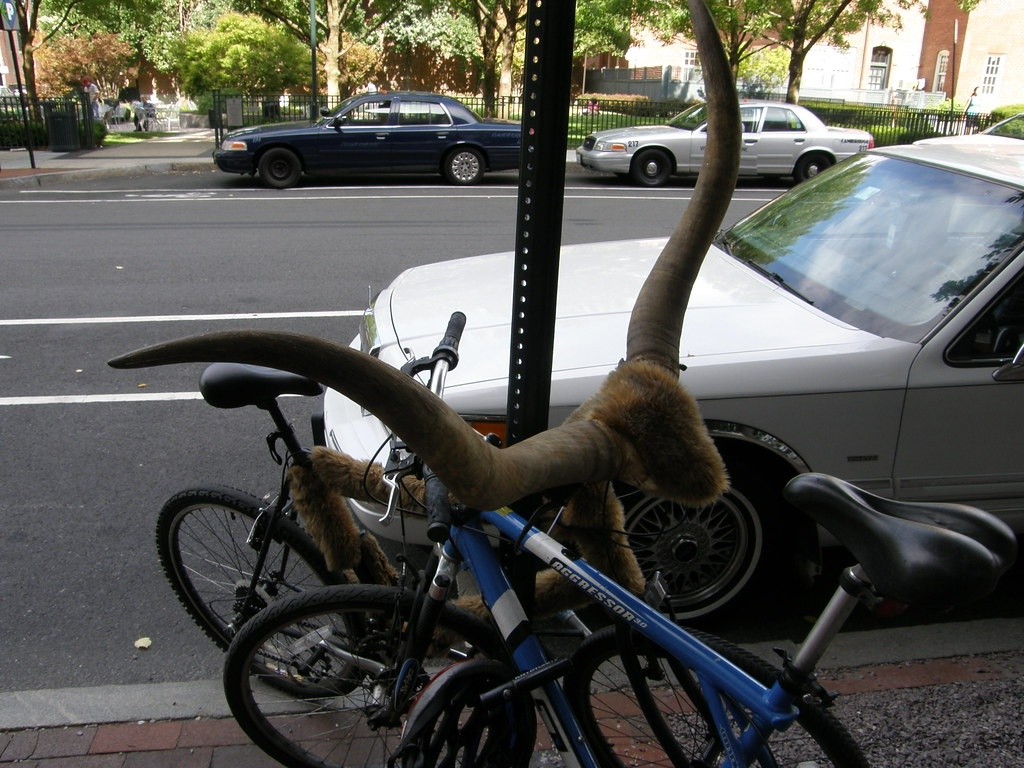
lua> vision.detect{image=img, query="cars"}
[574,99,875,188]
[310,132,1024,592]
[212,90,521,189]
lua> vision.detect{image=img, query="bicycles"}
[157,305,1024,768]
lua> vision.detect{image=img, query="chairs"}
[101,99,182,132]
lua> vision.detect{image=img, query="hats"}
[379,101,390,109]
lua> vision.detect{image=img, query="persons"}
[83,79,157,133]
[964,88,982,136]
